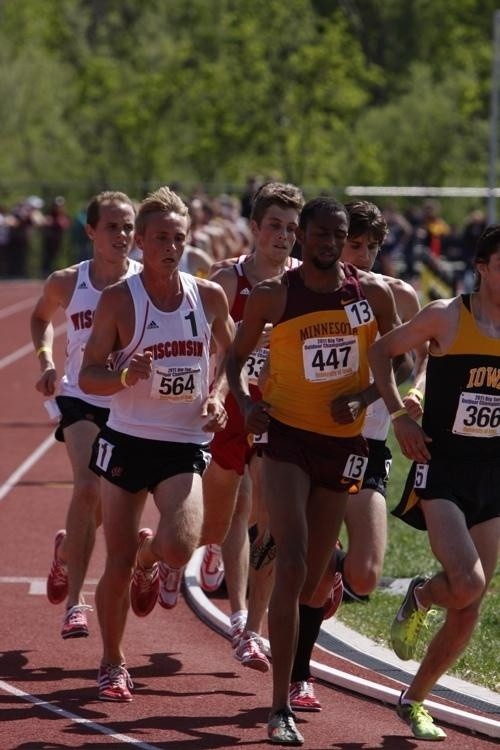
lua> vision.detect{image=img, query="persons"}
[2,193,92,281]
[28,182,435,709]
[372,198,487,301]
[221,195,415,745]
[365,212,500,746]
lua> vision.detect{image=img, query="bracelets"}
[386,408,410,422]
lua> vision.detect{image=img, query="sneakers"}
[96,657,133,702]
[200,528,278,672]
[322,538,343,619]
[396,688,447,741]
[390,574,432,660]
[268,712,304,745]
[290,675,322,711]
[47,528,186,639]
